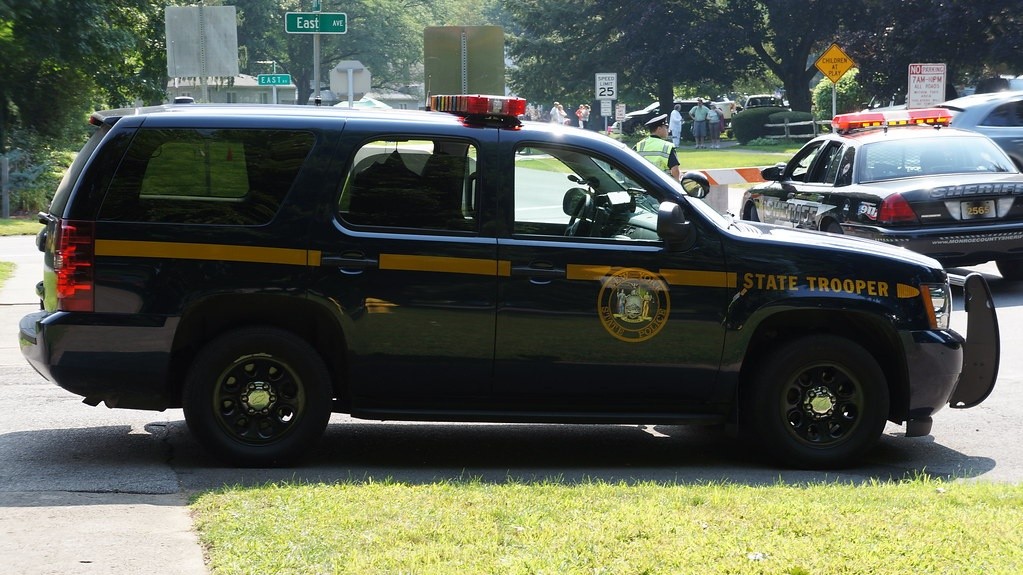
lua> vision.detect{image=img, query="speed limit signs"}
[595,73,617,100]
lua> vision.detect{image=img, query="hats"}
[710,102,717,107]
[643,114,668,126]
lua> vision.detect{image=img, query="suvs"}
[934,91,1023,174]
[19,94,1002,465]
[744,95,783,108]
[617,100,711,140]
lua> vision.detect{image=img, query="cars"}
[739,108,1023,278]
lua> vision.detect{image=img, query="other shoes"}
[710,145,715,149]
[696,145,699,149]
[701,145,706,148]
[715,145,719,148]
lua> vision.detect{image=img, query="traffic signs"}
[258,74,292,86]
[286,11,348,35]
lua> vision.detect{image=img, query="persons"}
[631,113,680,181]
[549,102,567,125]
[669,104,683,149]
[576,104,591,128]
[689,99,710,149]
[730,102,737,117]
[706,102,723,148]
